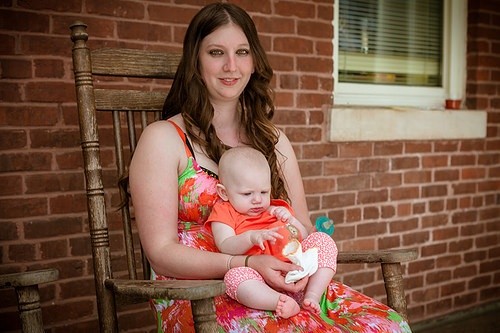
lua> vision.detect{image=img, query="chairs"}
[69,20,418,333]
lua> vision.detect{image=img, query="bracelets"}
[227,256,233,270]
[245,255,253,267]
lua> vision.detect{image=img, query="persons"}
[128,2,412,333]
[204,145,338,319]
